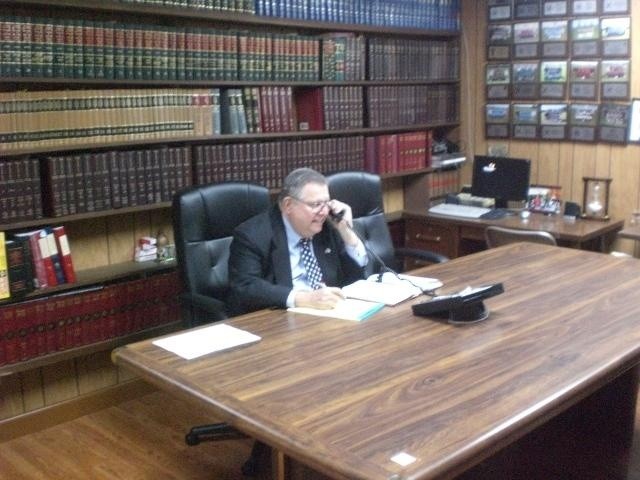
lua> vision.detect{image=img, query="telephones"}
[330,211,343,222]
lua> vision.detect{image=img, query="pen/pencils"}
[317,281,347,301]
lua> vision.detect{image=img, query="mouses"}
[520,210,531,218]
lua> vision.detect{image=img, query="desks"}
[618,227,640,257]
[401,204,624,276]
[111,243,640,480]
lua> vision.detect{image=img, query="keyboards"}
[427,202,492,219]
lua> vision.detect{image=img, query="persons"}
[224,166,372,319]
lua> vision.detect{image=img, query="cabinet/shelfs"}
[483,11,631,128]
[0,0,463,376]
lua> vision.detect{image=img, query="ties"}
[302,238,326,291]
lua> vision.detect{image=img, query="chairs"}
[327,171,449,271]
[172,183,272,479]
[485,227,556,254]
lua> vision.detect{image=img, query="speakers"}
[447,192,459,204]
[564,201,581,220]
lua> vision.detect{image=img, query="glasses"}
[284,192,329,211]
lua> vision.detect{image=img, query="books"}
[1,227,78,299]
[0,2,461,227]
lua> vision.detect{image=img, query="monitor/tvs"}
[471,155,530,216]
